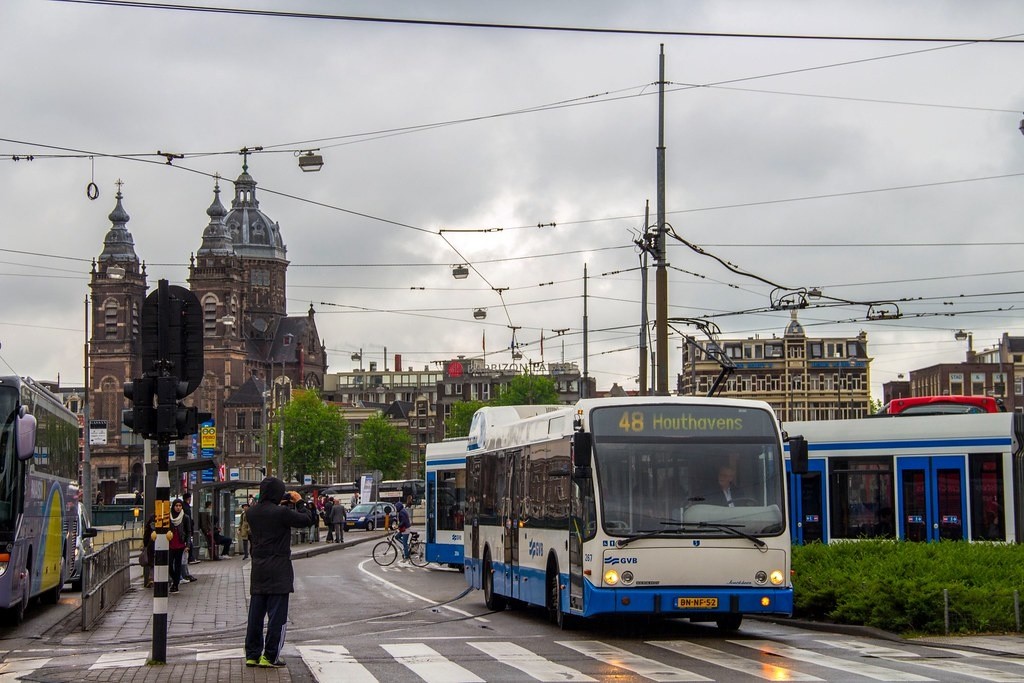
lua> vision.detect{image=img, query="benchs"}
[204,541,238,559]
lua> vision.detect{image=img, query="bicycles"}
[373,528,430,567]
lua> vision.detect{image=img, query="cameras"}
[281,493,291,500]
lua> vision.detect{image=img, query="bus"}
[113,494,183,507]
[464,398,808,630]
[759,411,1024,546]
[233,481,262,505]
[863,395,1002,530]
[425,438,466,573]
[285,479,425,512]
[0,375,81,627]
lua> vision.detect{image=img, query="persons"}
[406,495,413,527]
[394,502,411,563]
[703,466,756,506]
[245,477,312,668]
[96,490,252,593]
[281,489,360,545]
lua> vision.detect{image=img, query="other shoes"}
[180,578,190,583]
[219,555,233,559]
[146,578,154,588]
[309,540,319,544]
[334,539,344,543]
[184,575,197,582]
[189,559,202,564]
[213,557,223,561]
[169,586,180,594]
[243,557,248,560]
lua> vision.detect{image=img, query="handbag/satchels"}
[399,526,406,532]
[139,547,148,566]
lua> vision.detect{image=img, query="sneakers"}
[258,656,287,668]
[246,659,260,667]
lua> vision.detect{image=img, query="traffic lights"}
[173,380,190,435]
[123,378,151,437]
[258,468,265,475]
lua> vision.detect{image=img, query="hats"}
[242,503,250,508]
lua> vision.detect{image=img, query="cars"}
[343,502,398,532]
[64,502,97,591]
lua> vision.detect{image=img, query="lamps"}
[106,264,125,279]
[453,265,470,279]
[299,151,324,172]
[513,349,523,360]
[352,353,361,361]
[1019,112,1024,135]
[955,330,967,340]
[808,287,822,300]
[473,308,486,321]
